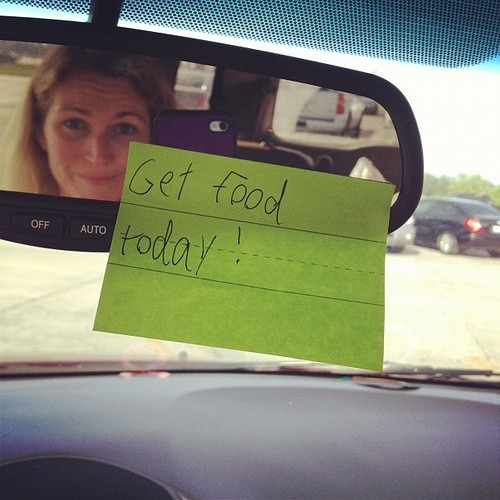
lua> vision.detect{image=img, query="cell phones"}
[154,108,235,157]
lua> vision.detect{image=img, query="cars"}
[413,196,500,258]
[385,192,417,254]
[293,87,368,140]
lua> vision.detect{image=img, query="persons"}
[3,44,176,205]
